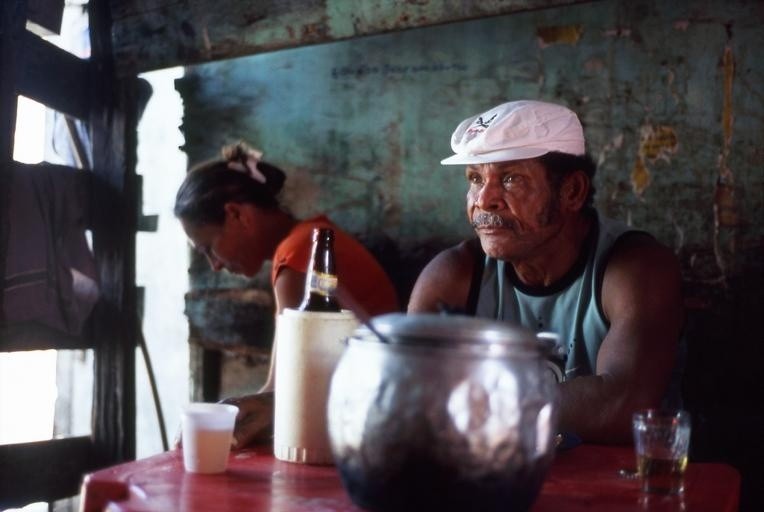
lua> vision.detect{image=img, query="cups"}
[632,408,690,497]
[180,403,239,475]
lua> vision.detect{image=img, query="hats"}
[440,100,584,166]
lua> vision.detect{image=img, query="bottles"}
[296,226,341,313]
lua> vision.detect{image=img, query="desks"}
[79,438,743,511]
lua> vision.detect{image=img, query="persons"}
[174,97,688,452]
[173,139,403,394]
